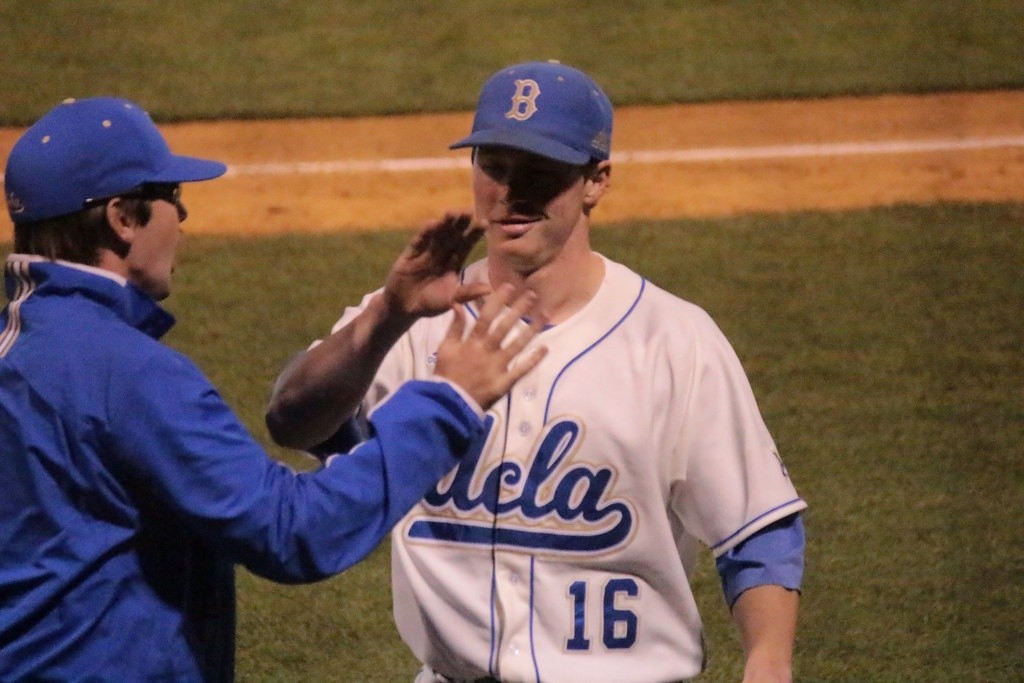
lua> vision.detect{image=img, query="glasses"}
[84,181,180,206]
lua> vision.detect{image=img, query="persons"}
[0,99,547,683]
[266,60,807,683]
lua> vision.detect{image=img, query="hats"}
[4,98,227,222]
[449,59,613,165]
